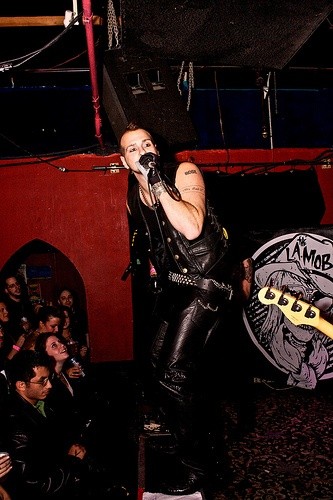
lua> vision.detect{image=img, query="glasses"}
[9,282,19,288]
[24,373,54,388]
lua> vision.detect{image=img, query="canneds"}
[21,316,28,324]
[70,358,86,380]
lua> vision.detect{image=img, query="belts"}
[167,271,217,292]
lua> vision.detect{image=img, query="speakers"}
[101,47,200,152]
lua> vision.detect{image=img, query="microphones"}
[145,160,158,168]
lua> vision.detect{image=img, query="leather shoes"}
[158,464,233,494]
